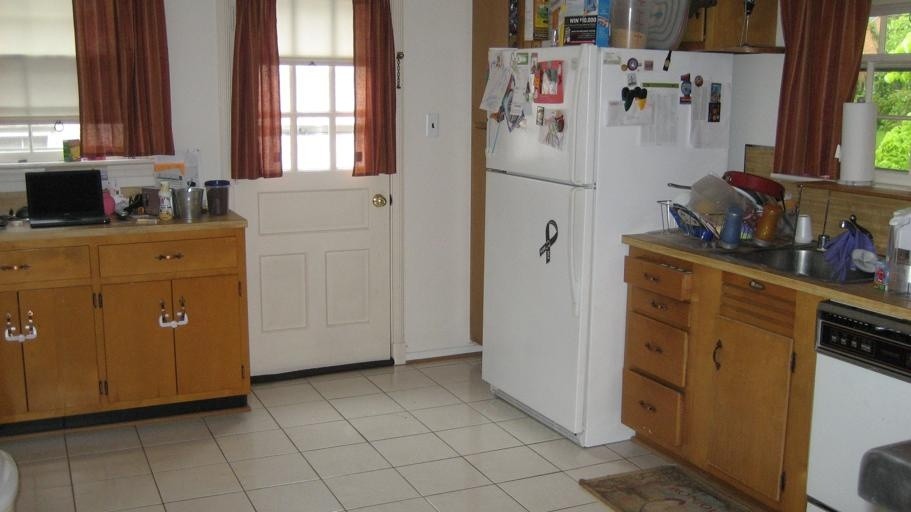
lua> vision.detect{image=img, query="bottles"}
[719,205,744,248]
[751,203,782,248]
[794,215,814,244]
[609,0,651,48]
[157,181,174,222]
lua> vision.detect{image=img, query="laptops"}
[24,169,110,228]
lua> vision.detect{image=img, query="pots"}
[667,171,785,201]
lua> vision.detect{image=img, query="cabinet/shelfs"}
[706,313,794,504]
[619,253,695,450]
[1,244,101,417]
[97,236,245,405]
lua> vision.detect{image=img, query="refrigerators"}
[480,47,734,447]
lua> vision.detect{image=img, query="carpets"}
[578,463,754,512]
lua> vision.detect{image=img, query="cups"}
[175,188,204,220]
[204,180,230,217]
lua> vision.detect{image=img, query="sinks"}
[711,242,874,284]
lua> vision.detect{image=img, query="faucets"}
[838,215,874,247]
